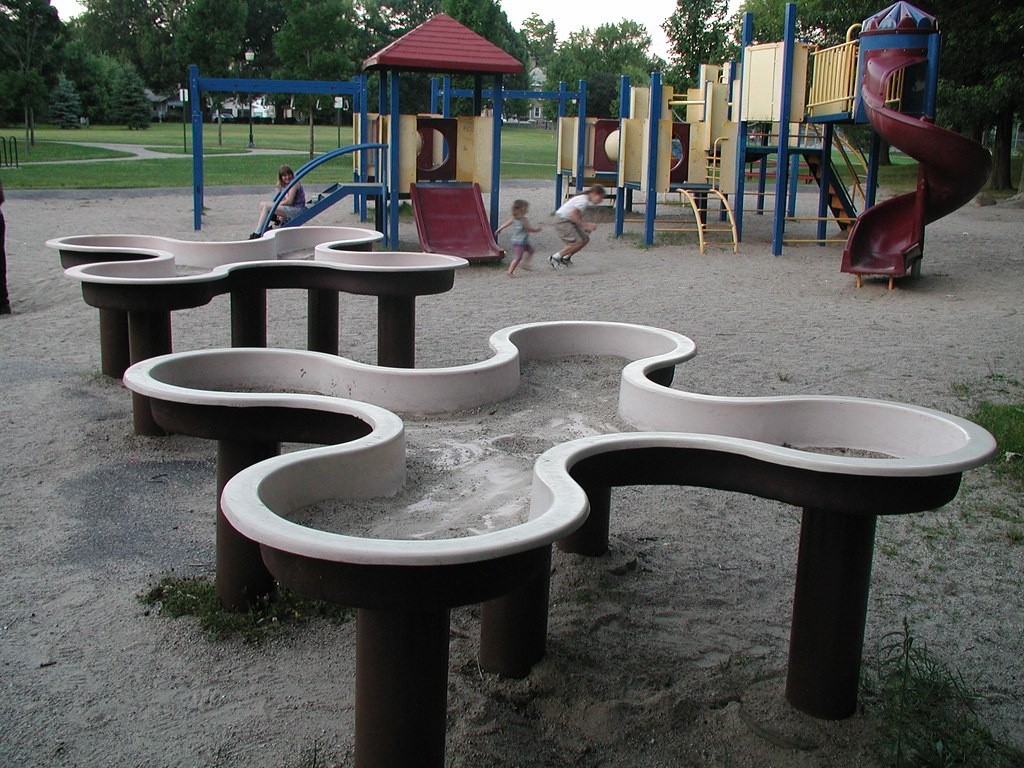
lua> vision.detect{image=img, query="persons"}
[248,165,306,241]
[547,184,606,271]
[496,199,543,279]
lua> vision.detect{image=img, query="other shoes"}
[523,265,533,271]
[248,232,261,240]
[270,223,280,229]
[506,271,516,277]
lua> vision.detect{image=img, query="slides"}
[409,181,506,263]
[839,84,994,278]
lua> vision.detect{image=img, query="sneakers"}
[547,256,568,270]
[561,257,573,267]
